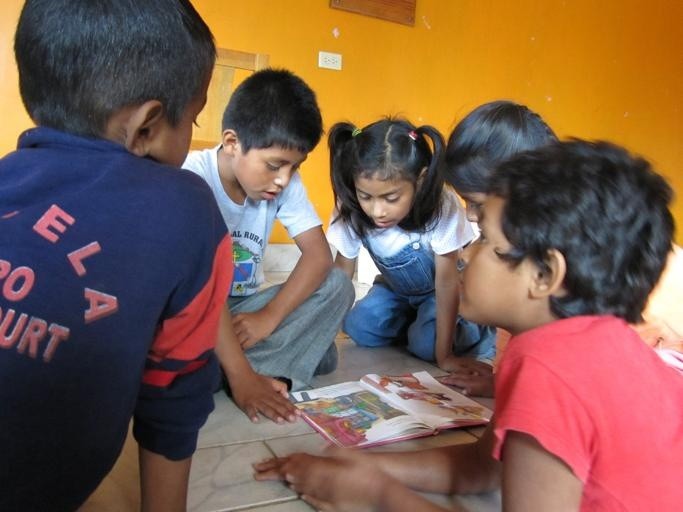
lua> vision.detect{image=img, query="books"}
[283,368,495,450]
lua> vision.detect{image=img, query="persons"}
[0,1,235,512]
[251,136,682,512]
[321,114,494,376]
[181,71,354,424]
[441,100,682,399]
